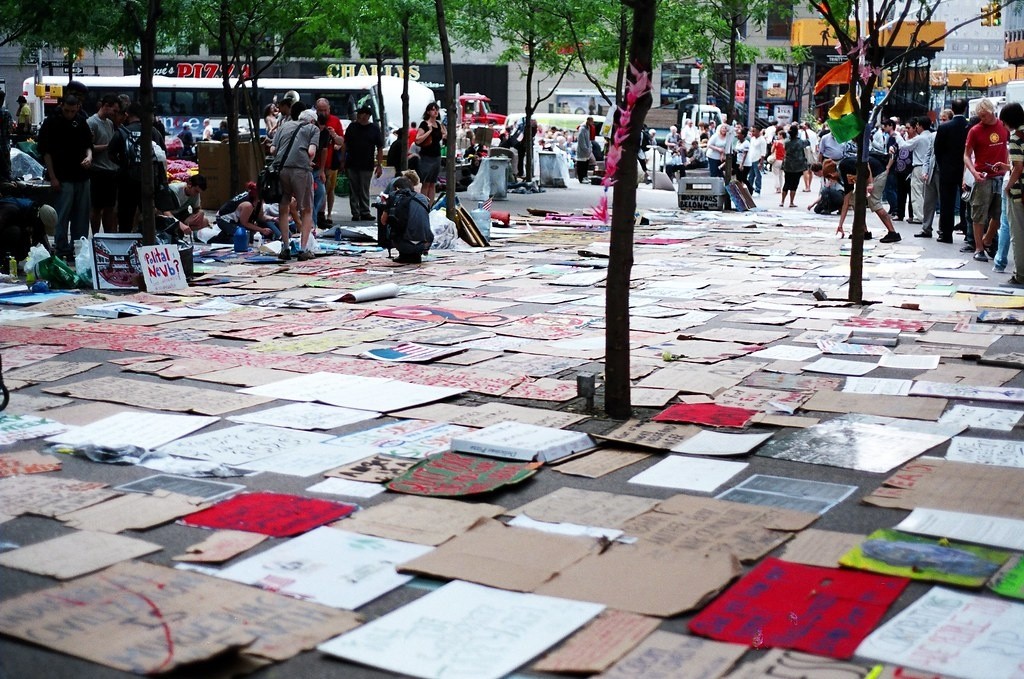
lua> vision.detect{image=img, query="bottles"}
[25,251,34,282]
[253,231,261,248]
[9,256,17,279]
[335,228,341,241]
[470,202,491,242]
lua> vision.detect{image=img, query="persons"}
[575,117,593,182]
[381,103,447,258]
[807,98,1024,286]
[213,97,384,261]
[462,120,593,183]
[638,116,818,206]
[0,81,209,275]
[511,107,537,178]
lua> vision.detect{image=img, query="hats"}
[356,104,372,116]
[39,205,58,228]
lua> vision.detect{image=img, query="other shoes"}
[297,249,316,261]
[360,213,376,221]
[747,187,1024,289]
[393,250,422,264]
[278,249,291,260]
[351,215,360,221]
[326,218,332,225]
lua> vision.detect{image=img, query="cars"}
[460,93,506,124]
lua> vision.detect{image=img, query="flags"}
[827,114,868,143]
[812,60,852,95]
[828,91,854,120]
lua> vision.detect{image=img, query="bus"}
[22,76,386,162]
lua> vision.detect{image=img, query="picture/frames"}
[92,237,145,290]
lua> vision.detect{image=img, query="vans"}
[505,114,606,153]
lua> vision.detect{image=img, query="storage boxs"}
[196,140,265,211]
[677,177,725,211]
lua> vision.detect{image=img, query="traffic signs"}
[64,67,84,74]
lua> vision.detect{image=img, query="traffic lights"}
[882,68,891,88]
[993,3,1001,27]
[980,5,990,27]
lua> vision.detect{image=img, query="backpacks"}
[118,125,159,187]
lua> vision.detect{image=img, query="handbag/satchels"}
[415,121,433,147]
[259,170,283,205]
[371,199,410,250]
[334,168,351,197]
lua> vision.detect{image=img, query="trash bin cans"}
[539,153,556,185]
[482,156,509,201]
